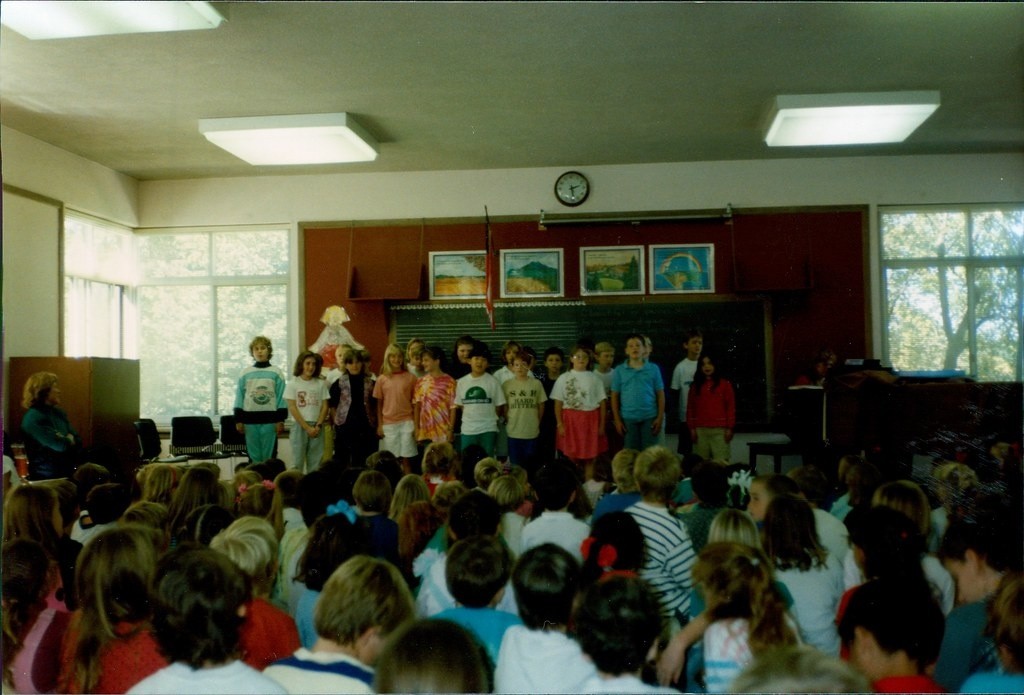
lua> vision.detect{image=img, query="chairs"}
[746,389,826,475]
[134,416,275,464]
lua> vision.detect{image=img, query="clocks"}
[554,171,590,207]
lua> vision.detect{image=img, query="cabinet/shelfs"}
[9,357,139,472]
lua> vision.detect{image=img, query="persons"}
[289,329,736,476]
[655,541,804,694]
[259,501,416,695]
[233,336,287,464]
[124,541,289,695]
[2,441,1024,594]
[371,617,495,695]
[206,513,303,672]
[57,528,171,695]
[21,371,83,478]
[733,646,868,694]
[932,516,1024,694]
[797,349,836,393]
[494,542,683,695]
[0,537,74,695]
[837,577,948,694]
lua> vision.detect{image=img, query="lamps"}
[759,90,942,147]
[199,112,380,166]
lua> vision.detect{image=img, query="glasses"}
[573,355,590,361]
